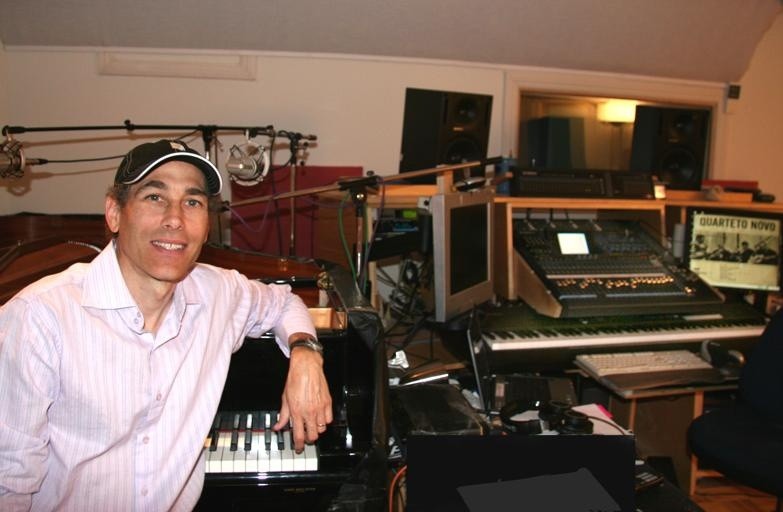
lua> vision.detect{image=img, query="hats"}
[114,139,222,196]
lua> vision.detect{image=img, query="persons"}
[690,233,710,261]
[733,241,755,264]
[0,136,336,512]
[710,243,733,262]
[753,241,779,266]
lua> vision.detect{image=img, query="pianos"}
[0,233,390,511]
[477,295,768,352]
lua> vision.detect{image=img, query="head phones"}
[499,398,593,435]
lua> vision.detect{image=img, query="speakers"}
[398,87,493,191]
[629,104,709,191]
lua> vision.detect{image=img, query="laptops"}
[468,303,579,415]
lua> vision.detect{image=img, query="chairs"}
[685,309,781,510]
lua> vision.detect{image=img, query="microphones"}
[0,141,48,178]
[226,144,266,183]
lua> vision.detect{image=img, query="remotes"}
[634,469,665,494]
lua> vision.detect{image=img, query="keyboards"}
[576,349,713,377]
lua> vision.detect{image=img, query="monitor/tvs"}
[683,205,783,298]
[432,186,495,323]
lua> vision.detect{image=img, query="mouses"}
[727,349,745,364]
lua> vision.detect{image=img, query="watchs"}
[287,336,326,361]
